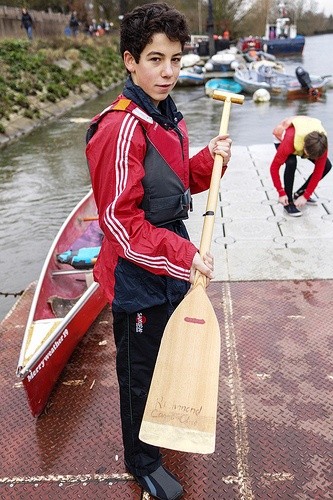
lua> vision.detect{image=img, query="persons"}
[86,1,232,500]
[270,116,332,217]
[87,19,110,36]
[20,6,35,40]
[69,14,80,40]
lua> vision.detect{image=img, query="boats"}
[16,185,108,421]
[175,1,305,88]
[231,48,333,104]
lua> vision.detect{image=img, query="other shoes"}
[135,465,184,500]
[283,203,302,217]
[293,193,318,205]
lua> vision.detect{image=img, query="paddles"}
[138,89,244,455]
